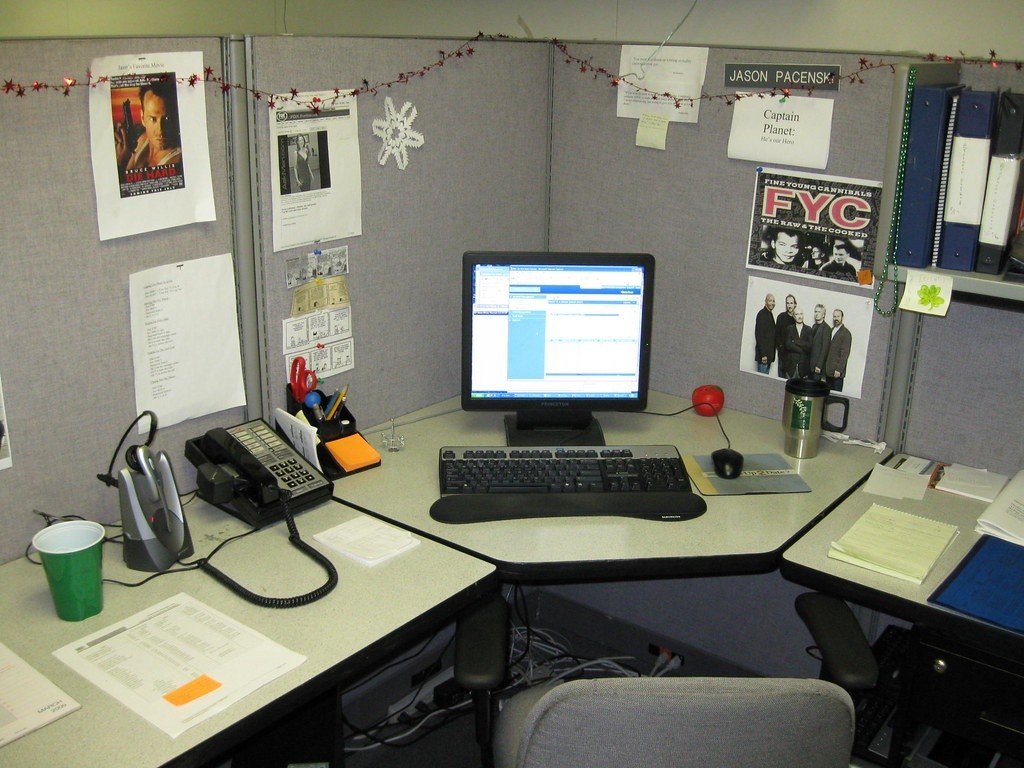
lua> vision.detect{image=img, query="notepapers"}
[325,433,381,473]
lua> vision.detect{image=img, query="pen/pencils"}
[313,384,349,421]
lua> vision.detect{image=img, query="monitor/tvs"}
[461,251,655,446]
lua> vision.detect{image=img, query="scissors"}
[289,356,317,404]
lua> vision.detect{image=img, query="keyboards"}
[430,445,707,525]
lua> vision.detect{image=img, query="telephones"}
[184,416,334,531]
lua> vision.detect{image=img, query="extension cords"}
[387,666,456,726]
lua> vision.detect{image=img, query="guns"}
[118,98,138,154]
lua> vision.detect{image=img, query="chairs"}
[458,591,886,768]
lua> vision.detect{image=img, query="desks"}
[0,387,1024,768]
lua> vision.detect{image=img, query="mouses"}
[711,448,744,479]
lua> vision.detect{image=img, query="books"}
[935,462,1024,546]
[896,83,1024,273]
[314,515,419,567]
[828,503,959,584]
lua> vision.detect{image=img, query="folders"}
[892,82,1024,275]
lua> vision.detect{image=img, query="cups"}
[782,378,850,459]
[32,520,107,621]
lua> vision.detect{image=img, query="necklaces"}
[875,68,916,316]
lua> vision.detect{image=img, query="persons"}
[113,73,182,170]
[753,227,862,392]
[293,134,315,191]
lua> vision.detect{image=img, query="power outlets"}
[641,640,685,669]
